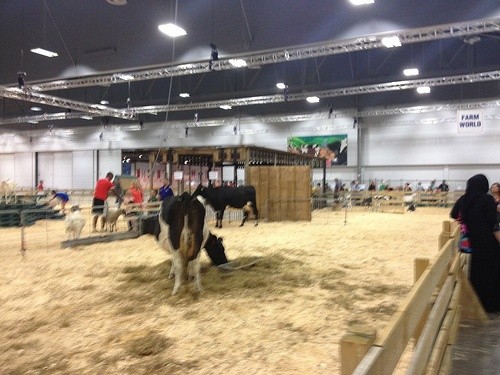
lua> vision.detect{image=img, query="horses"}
[192,183,259,227]
[155,191,228,300]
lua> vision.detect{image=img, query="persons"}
[38,172,500,320]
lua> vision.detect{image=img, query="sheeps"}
[66,203,86,240]
[101,209,126,233]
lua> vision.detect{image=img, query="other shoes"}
[91,229,98,233]
[100,228,106,233]
